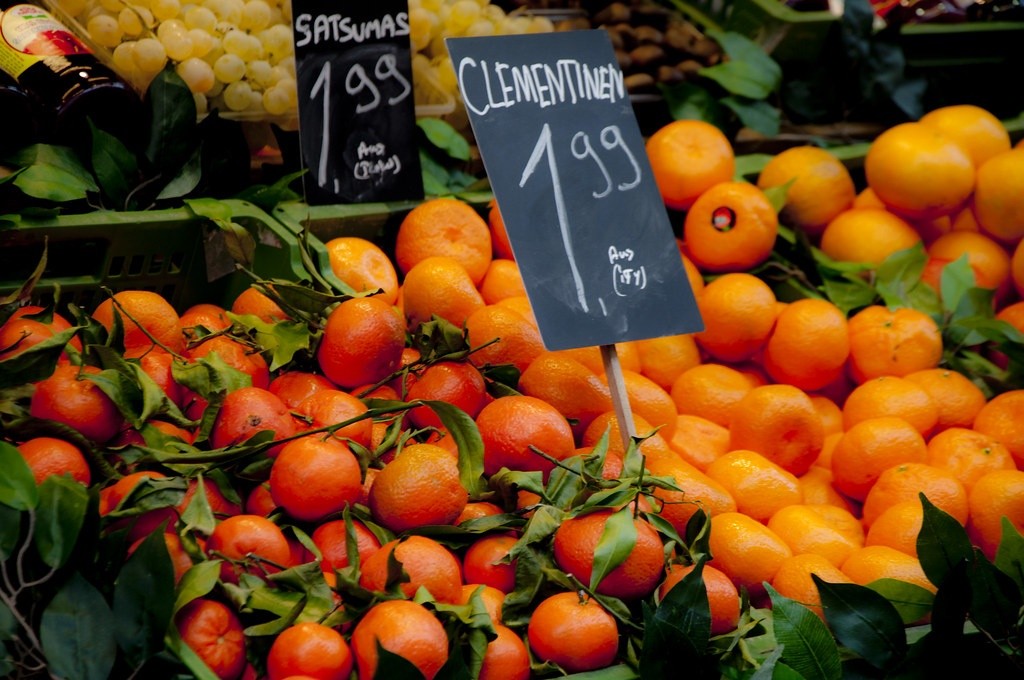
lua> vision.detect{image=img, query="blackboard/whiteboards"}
[447,26,707,352]
[291,0,426,209]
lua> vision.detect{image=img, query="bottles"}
[0,0,151,166]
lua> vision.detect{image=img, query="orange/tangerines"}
[0,104,1023,680]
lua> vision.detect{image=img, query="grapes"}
[53,0,554,115]
